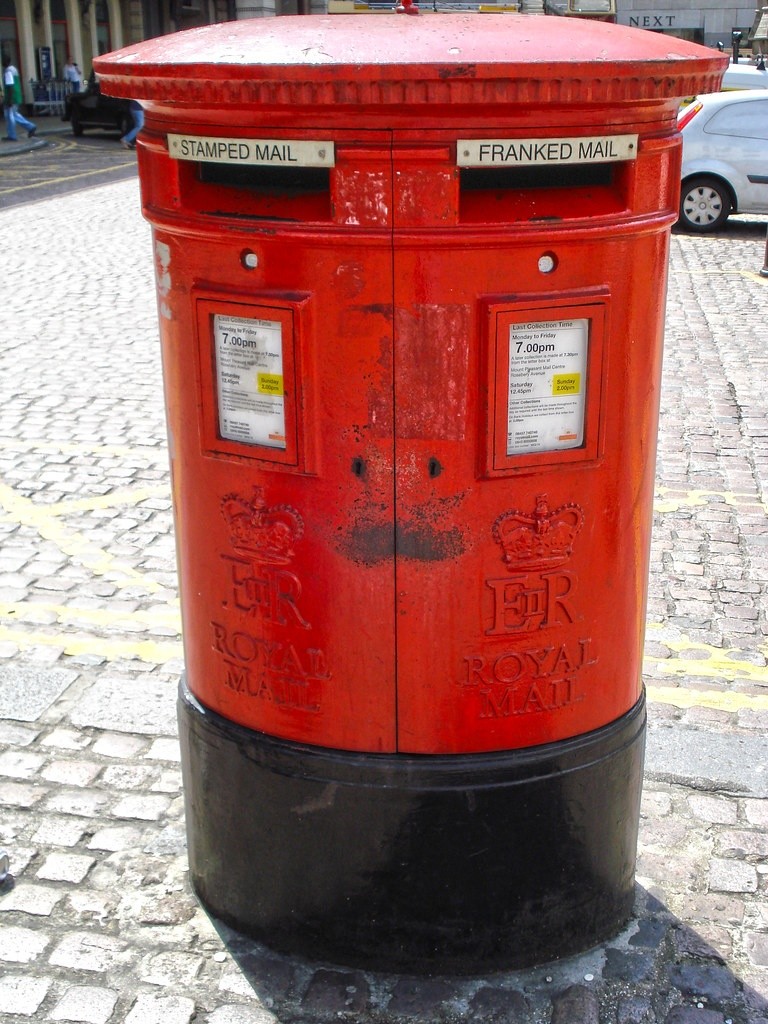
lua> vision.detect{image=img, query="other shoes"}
[120,138,132,147]
[2,137,16,141]
[29,128,36,138]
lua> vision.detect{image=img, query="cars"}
[62,66,134,137]
[676,89,768,232]
[720,56,768,91]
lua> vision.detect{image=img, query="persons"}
[120,99,144,149]
[2,55,37,141]
[62,56,81,91]
